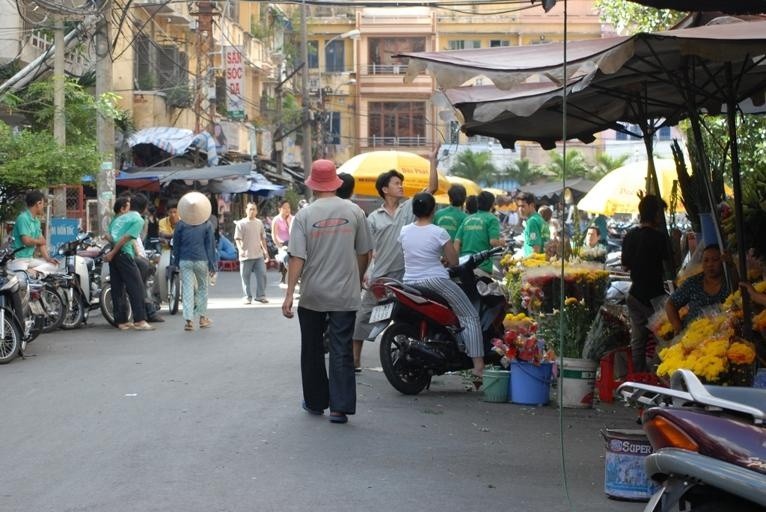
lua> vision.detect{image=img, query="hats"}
[304,159,344,192]
[178,192,212,225]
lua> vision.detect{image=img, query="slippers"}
[119,315,164,329]
[329,414,347,420]
[184,323,193,329]
[200,318,212,326]
[255,298,268,303]
[243,300,251,303]
[303,402,325,415]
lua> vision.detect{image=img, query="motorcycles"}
[1,235,185,362]
[366,245,512,396]
[616,366,766,510]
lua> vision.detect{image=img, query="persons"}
[297,199,306,213]
[7,191,61,292]
[316,172,364,356]
[279,158,372,426]
[398,184,605,395]
[271,199,294,290]
[622,195,734,385]
[90,190,238,334]
[232,201,270,304]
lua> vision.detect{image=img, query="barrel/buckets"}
[479,369,511,403]
[555,357,597,407]
[600,429,662,500]
[510,359,554,405]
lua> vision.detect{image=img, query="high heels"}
[472,375,483,391]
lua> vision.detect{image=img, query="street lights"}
[318,28,364,148]
[327,73,360,143]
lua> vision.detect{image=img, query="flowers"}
[655,257,766,385]
[489,243,613,371]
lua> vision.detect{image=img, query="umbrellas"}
[348,141,450,374]
[332,150,452,201]
